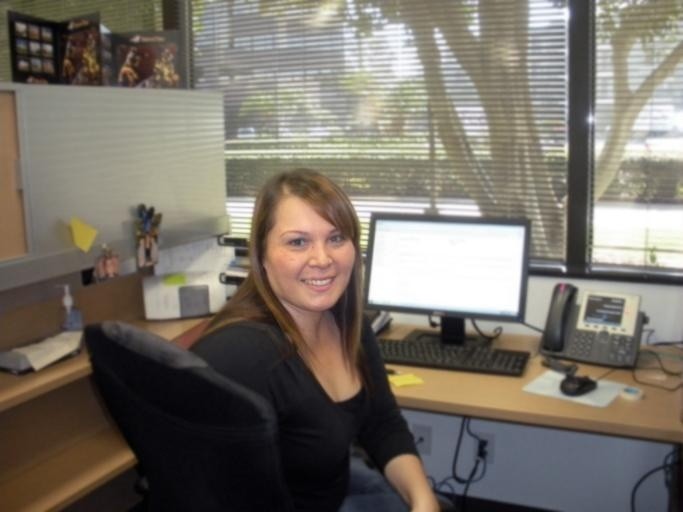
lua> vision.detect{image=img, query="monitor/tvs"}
[361,211,532,344]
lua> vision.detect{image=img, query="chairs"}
[84,320,282,511]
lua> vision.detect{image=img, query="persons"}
[137,232,160,268]
[184,166,460,512]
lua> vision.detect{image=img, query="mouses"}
[560,374,596,396]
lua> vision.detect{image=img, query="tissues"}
[139,236,235,323]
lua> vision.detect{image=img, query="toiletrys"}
[58,282,84,332]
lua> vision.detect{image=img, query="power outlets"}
[411,423,432,456]
[474,434,495,464]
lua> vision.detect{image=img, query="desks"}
[370,324,683,447]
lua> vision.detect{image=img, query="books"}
[218,232,251,303]
[363,308,394,334]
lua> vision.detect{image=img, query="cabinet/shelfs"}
[0,317,209,512]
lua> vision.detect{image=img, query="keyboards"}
[375,336,530,376]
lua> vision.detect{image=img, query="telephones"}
[539,283,644,367]
